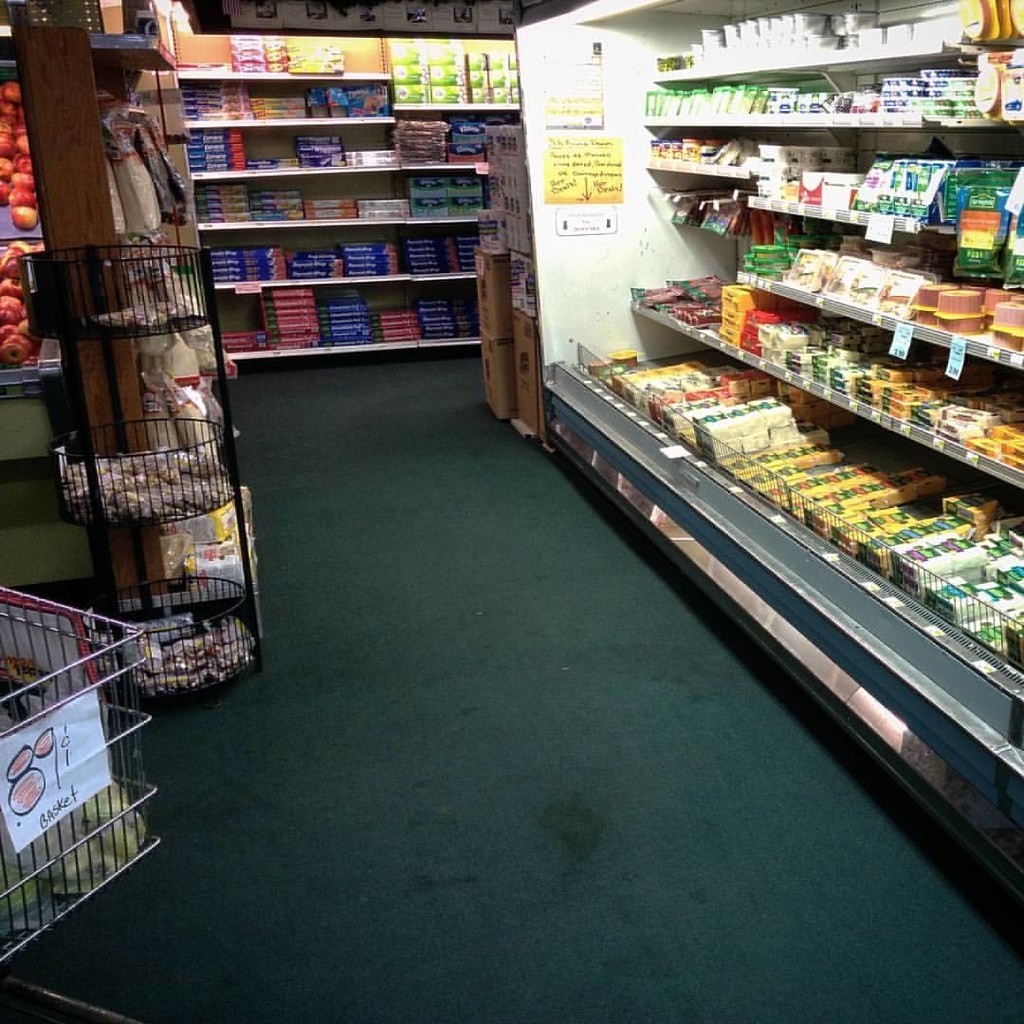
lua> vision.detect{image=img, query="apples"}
[0,241,45,365]
[0,82,38,229]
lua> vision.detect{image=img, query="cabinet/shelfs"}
[571,35,1024,691]
[17,247,263,701]
[13,25,260,609]
[175,36,522,360]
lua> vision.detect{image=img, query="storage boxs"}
[181,36,546,445]
[590,67,1024,668]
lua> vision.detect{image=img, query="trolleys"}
[0,581,170,1024]
